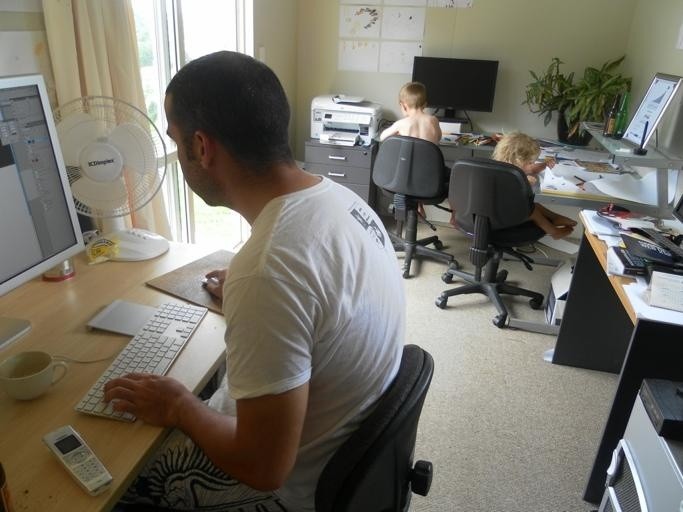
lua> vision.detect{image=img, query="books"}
[609,246,645,275]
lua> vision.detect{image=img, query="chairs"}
[370,134,460,281]
[434,157,545,328]
[313,341,435,511]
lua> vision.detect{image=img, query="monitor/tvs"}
[0,72,86,349]
[412,56,499,124]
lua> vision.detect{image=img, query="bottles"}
[603,87,631,140]
[41,256,77,282]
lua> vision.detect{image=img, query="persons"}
[491,130,577,240]
[103,51,406,512]
[379,82,442,220]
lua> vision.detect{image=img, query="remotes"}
[641,227,683,259]
[42,425,113,497]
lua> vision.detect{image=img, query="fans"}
[51,93,171,264]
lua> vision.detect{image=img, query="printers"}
[310,95,382,146]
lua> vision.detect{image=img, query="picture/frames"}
[619,71,682,150]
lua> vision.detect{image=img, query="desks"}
[372,119,681,254]
[552,207,682,505]
[0,242,226,511]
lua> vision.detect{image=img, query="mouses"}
[201,277,219,288]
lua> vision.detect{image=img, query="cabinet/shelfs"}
[304,139,376,209]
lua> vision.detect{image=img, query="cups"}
[1,352,67,400]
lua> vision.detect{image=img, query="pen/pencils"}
[640,121,649,149]
[575,176,586,183]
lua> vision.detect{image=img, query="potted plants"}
[518,52,627,147]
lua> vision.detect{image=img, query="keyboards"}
[72,303,208,422]
